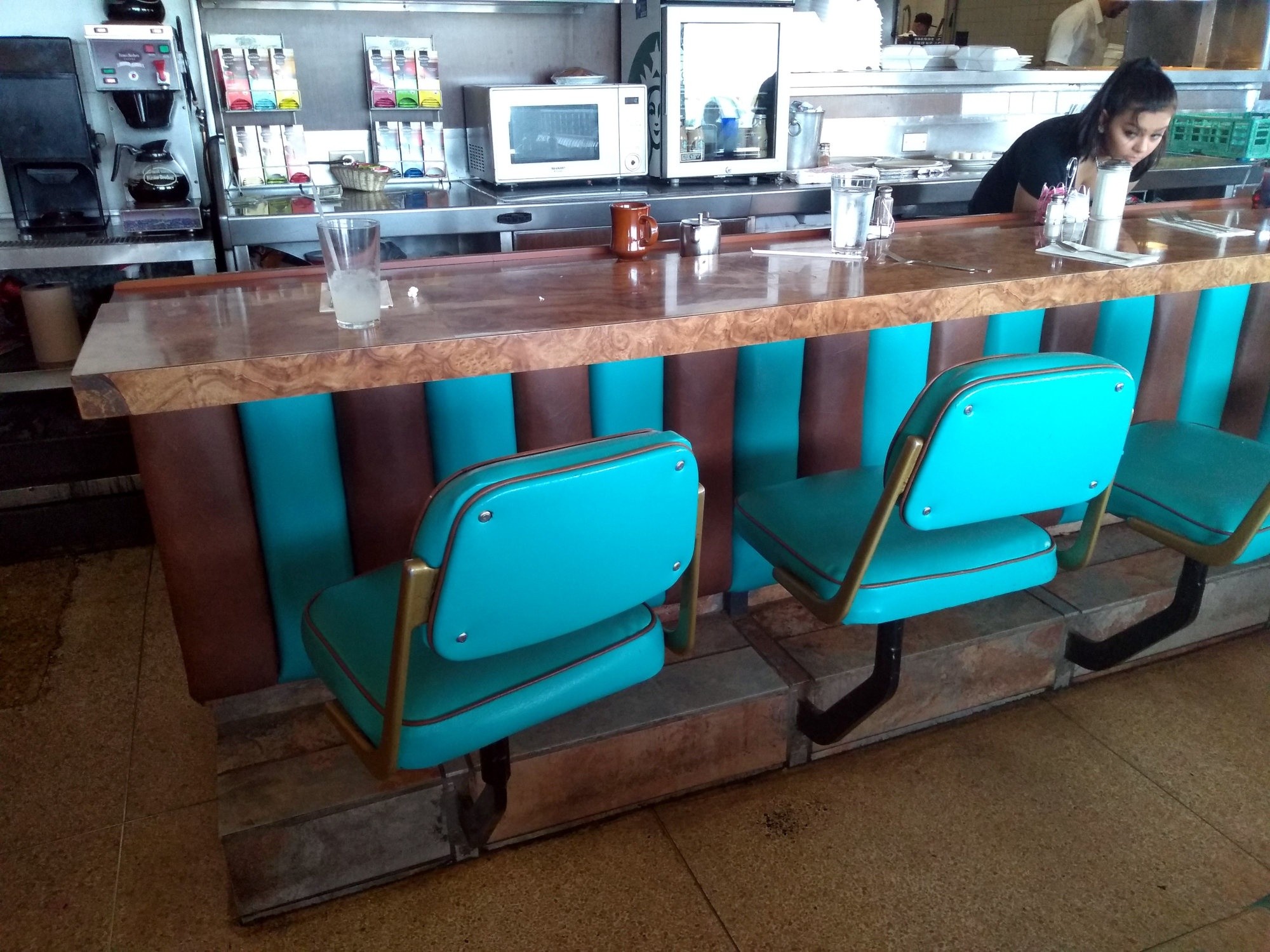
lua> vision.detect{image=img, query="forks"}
[1160,210,1231,231]
[881,249,993,273]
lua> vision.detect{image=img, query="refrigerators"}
[617,1,790,187]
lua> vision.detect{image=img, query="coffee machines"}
[0,34,110,236]
[83,19,206,233]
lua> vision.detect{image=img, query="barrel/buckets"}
[788,111,826,173]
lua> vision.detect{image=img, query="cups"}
[316,218,383,329]
[609,202,659,262]
[829,174,878,256]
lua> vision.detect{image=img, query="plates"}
[550,74,609,86]
[829,149,996,173]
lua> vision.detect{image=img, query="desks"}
[71,199,1270,930]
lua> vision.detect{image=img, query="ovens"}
[463,83,649,188]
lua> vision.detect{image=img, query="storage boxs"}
[1163,111,1270,163]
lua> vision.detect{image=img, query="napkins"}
[319,280,393,312]
[1146,216,1256,238]
[1035,239,1159,268]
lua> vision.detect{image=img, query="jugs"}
[110,139,191,203]
[678,210,721,257]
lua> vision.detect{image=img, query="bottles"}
[1086,219,1124,252]
[1091,160,1134,220]
[676,112,771,162]
[1044,193,1065,225]
[818,143,830,168]
[869,185,896,238]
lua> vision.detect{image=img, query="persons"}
[897,12,938,45]
[966,55,1178,212]
[1044,0,1131,66]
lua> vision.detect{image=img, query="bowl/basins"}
[877,42,1033,71]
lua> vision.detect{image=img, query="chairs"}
[300,428,709,842]
[1066,418,1269,668]
[733,351,1137,743]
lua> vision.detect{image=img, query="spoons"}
[1055,241,1130,261]
